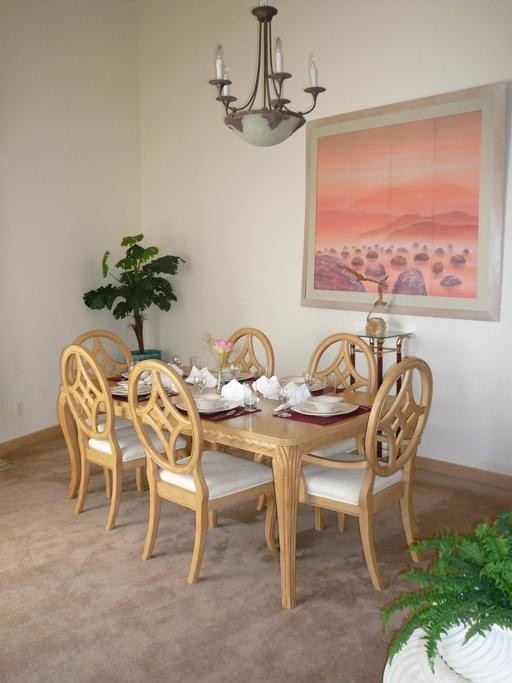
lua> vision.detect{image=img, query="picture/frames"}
[299,82,511,323]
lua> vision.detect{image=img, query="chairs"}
[256,333,377,534]
[127,361,284,582]
[294,356,434,591]
[188,329,273,527]
[73,329,148,497]
[60,346,188,530]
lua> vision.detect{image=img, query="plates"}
[291,402,361,417]
[176,403,241,415]
[110,386,152,397]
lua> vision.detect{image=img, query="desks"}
[79,367,407,610]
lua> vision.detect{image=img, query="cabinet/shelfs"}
[352,330,413,464]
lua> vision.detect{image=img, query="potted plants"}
[376,510,512,683]
[81,233,187,361]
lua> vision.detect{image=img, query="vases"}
[215,371,225,394]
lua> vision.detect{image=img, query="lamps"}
[209,0,326,149]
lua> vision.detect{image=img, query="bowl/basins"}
[118,379,145,389]
[194,396,221,406]
[307,396,344,410]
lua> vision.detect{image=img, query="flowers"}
[198,330,234,371]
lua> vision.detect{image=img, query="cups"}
[243,381,257,412]
[193,376,206,395]
[173,356,186,370]
[229,362,241,376]
[277,386,291,418]
[302,372,316,387]
[323,374,337,395]
[190,357,202,369]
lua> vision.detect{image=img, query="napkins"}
[145,362,183,389]
[273,383,314,411]
[222,379,260,408]
[184,364,218,389]
[250,374,283,400]
